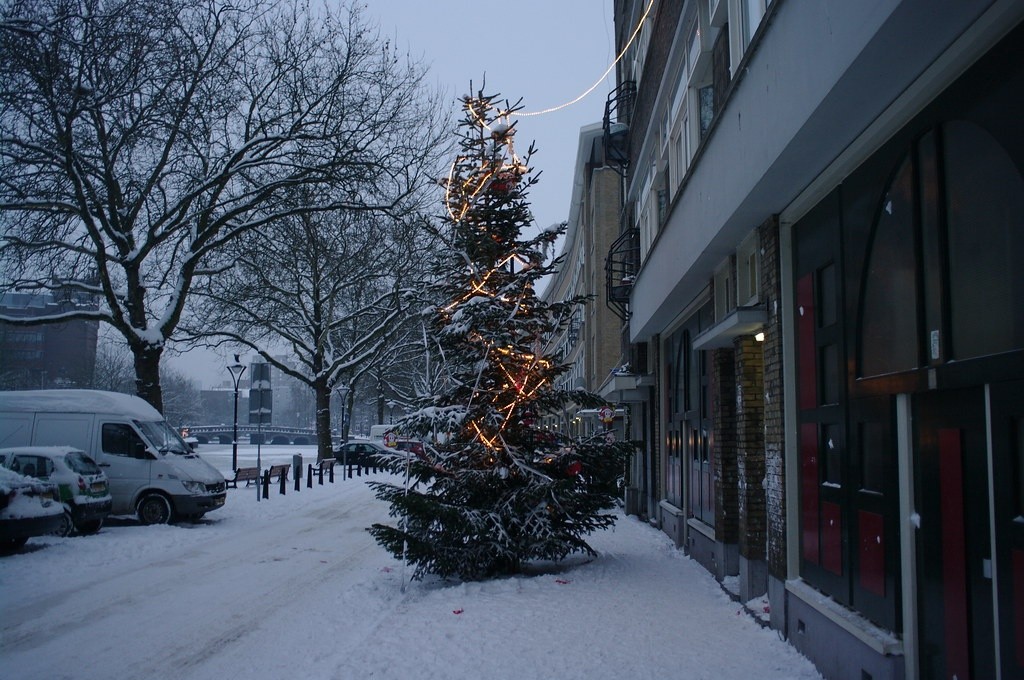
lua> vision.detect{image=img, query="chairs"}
[360,444,367,451]
[355,444,361,451]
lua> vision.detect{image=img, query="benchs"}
[312,458,337,476]
[224,467,258,488]
[260,464,291,484]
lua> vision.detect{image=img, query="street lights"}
[41,370,48,389]
[337,381,352,444]
[385,398,399,425]
[225,353,247,473]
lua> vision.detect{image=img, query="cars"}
[332,424,447,468]
[0,445,113,534]
[0,464,67,549]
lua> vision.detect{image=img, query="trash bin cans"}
[294,454,304,480]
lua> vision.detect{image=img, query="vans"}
[1,387,229,527]
